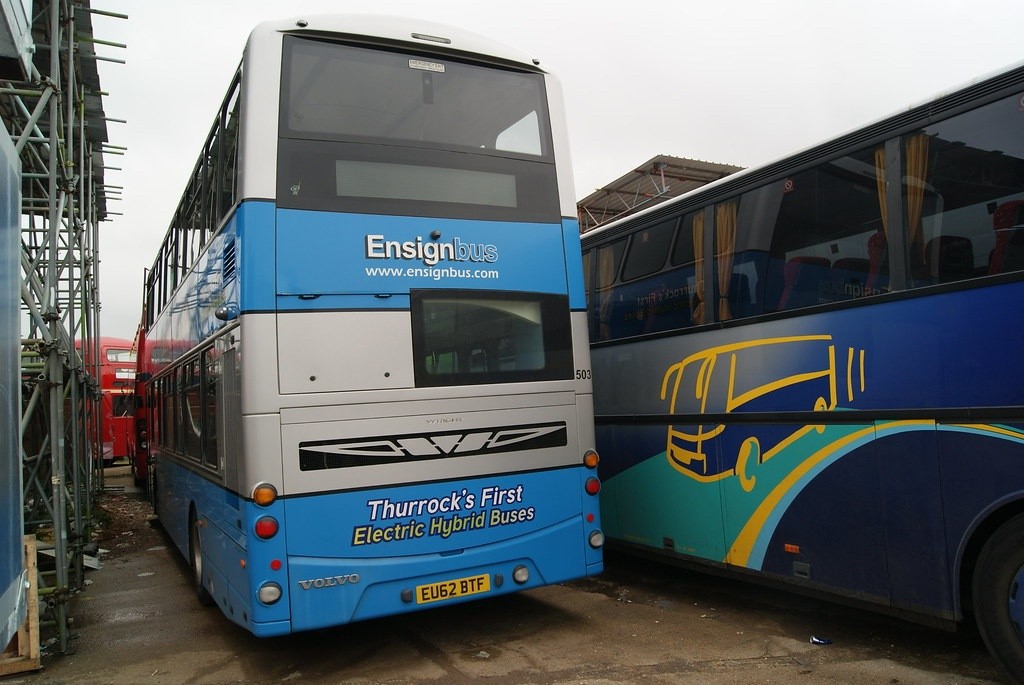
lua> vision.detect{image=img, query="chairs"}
[602,196,1024,335]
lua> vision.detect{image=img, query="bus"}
[580,63,1023,684]
[125,339,239,462]
[74,336,137,468]
[114,23,605,642]
